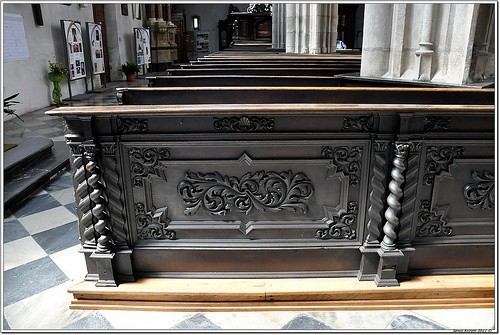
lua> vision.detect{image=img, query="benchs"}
[43,103,495,309]
[114,85,496,105]
[145,47,425,87]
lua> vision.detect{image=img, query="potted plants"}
[118,62,142,82]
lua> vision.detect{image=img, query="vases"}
[48,73,65,106]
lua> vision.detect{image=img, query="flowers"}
[48,58,68,77]
